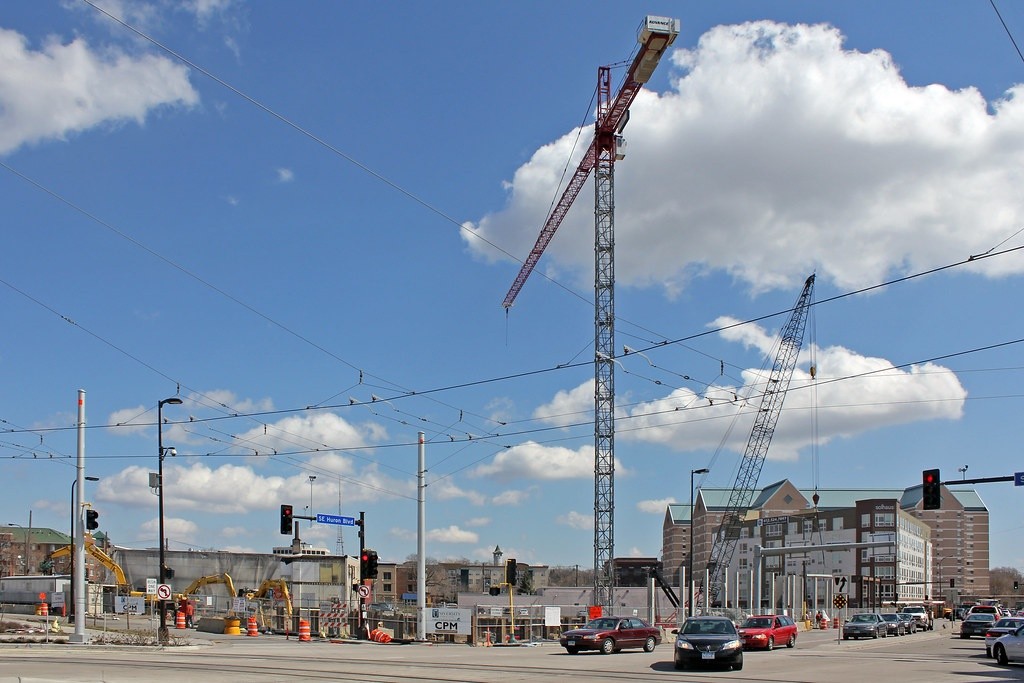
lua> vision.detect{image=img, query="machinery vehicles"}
[167,573,241,617]
[44,532,158,615]
[240,579,293,625]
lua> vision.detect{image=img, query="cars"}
[671,617,747,669]
[897,614,917,634]
[843,613,887,640]
[881,613,906,636]
[954,599,1024,665]
[560,616,661,655]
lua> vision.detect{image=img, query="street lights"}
[9,523,27,574]
[939,558,946,600]
[158,398,184,639]
[69,476,99,623]
[689,469,710,617]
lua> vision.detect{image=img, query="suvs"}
[734,615,798,651]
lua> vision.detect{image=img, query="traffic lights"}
[1014,581,1018,589]
[922,469,941,509]
[281,504,293,535]
[352,583,358,591]
[362,551,378,579]
[490,588,500,596]
[86,509,99,530]
[507,559,517,585]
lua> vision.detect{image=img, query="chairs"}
[715,624,720,630]
[755,620,768,625]
[607,623,613,627]
[1007,622,1016,627]
[690,622,701,634]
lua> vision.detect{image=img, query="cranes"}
[502,14,680,616]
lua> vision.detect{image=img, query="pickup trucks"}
[896,606,933,631]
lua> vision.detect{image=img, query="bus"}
[933,602,951,620]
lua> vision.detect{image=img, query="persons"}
[185,601,195,629]
[805,610,831,629]
